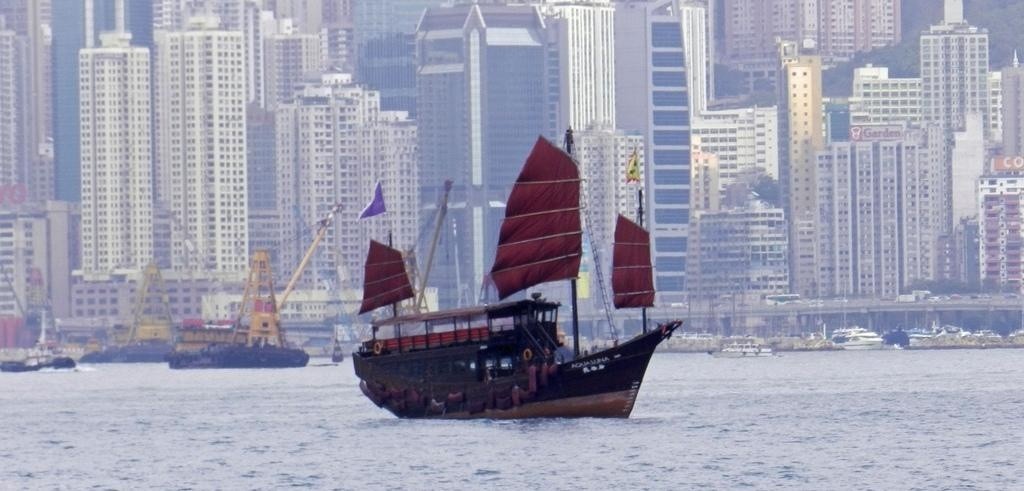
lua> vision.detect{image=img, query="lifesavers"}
[524,349,532,361]
[374,342,381,354]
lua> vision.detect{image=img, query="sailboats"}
[346,122,681,421]
[592,284,833,354]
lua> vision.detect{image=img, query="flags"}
[355,183,387,221]
[627,149,641,186]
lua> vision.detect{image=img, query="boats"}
[833,309,1024,351]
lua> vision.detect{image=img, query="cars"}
[893,289,1019,301]
